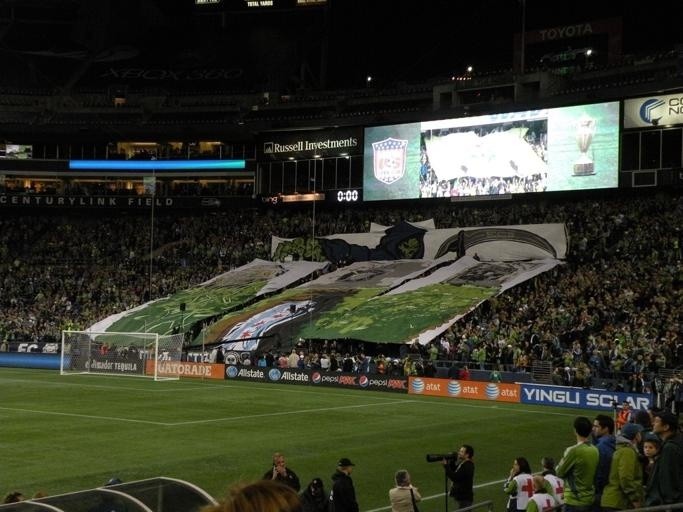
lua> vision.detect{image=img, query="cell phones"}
[272,462,276,469]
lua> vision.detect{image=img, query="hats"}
[338,458,355,466]
[651,411,678,432]
[622,423,643,439]
[628,409,651,428]
[311,477,323,488]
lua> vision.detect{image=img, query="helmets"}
[642,432,662,443]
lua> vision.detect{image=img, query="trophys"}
[571,113,597,177]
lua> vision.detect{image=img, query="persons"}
[502,374,683,511]
[331,457,359,512]
[0,148,683,374]
[389,469,421,512]
[264,451,300,490]
[443,442,475,511]
[301,477,330,512]
[3,491,26,504]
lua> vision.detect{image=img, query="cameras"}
[608,398,623,409]
